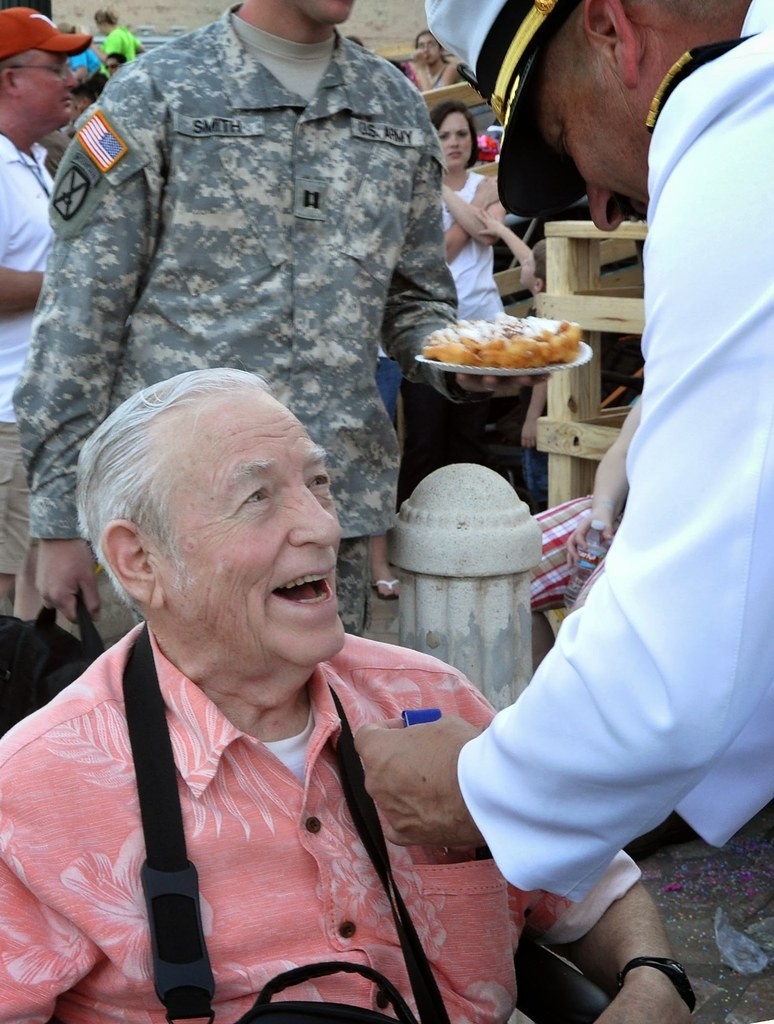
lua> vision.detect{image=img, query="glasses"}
[9,62,72,81]
[105,64,118,69]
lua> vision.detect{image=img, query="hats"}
[424,0,588,217]
[0,7,93,61]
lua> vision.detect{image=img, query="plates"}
[414,340,594,376]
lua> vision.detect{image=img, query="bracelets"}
[617,956,696,1013]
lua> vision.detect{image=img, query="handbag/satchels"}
[231,959,417,1023]
[0,594,105,739]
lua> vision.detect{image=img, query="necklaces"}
[354,0,774,903]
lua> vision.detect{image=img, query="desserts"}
[422,311,582,370]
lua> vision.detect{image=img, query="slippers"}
[371,579,400,600]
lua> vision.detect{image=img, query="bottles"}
[564,520,611,608]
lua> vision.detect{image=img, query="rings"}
[432,846,448,857]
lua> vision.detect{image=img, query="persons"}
[0,369,695,1023]
[36,7,145,182]
[0,7,94,738]
[565,397,644,568]
[345,31,460,93]
[476,208,548,512]
[396,99,505,516]
[12,0,548,634]
